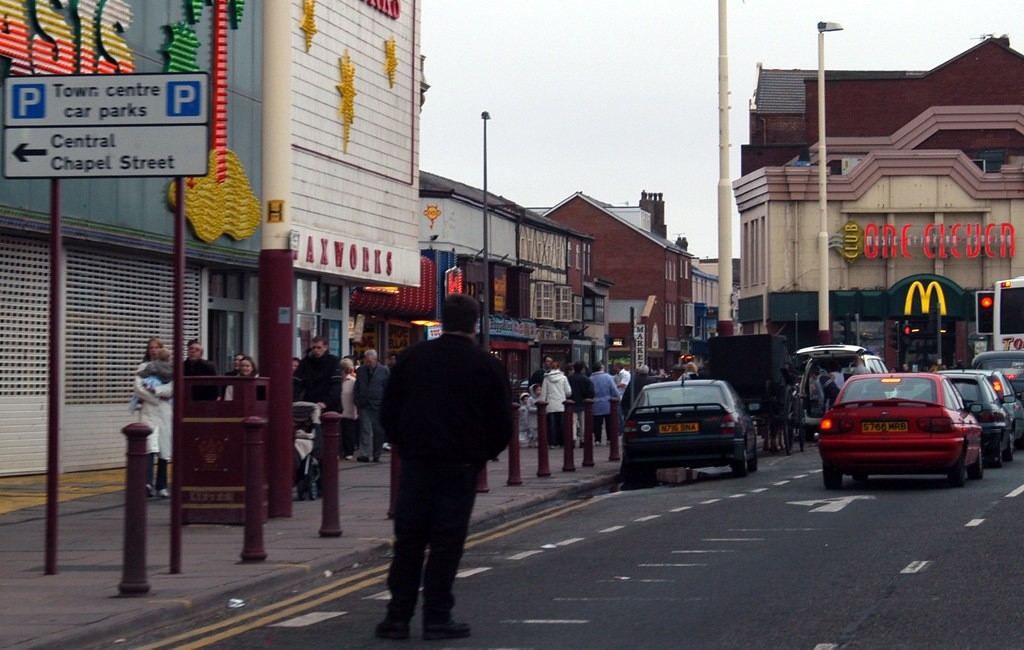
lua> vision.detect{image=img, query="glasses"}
[312,345,324,349]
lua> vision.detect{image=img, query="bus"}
[993,275,1024,351]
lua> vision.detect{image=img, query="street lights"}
[817,19,843,347]
[478,111,491,354]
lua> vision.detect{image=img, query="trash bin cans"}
[182,376,271,524]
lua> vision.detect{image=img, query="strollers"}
[291,400,321,502]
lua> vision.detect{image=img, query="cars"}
[922,348,1024,469]
[819,373,984,491]
[623,381,761,488]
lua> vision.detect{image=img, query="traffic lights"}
[974,290,994,336]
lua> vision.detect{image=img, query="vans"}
[799,346,890,442]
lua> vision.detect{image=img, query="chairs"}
[860,383,887,399]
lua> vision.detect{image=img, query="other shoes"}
[382,442,391,450]
[529,440,538,447]
[580,442,584,448]
[372,457,380,462]
[596,441,602,446]
[375,619,411,640]
[157,488,170,497]
[146,484,153,497]
[356,456,370,462]
[424,617,472,640]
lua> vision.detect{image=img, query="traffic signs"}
[4,125,206,180]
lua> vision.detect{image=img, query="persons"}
[889,356,967,375]
[133,332,400,498]
[805,355,875,421]
[372,292,514,640]
[517,355,707,447]
